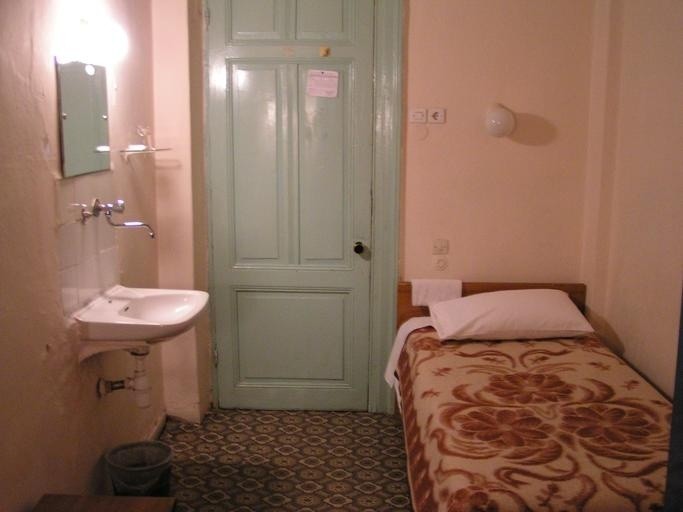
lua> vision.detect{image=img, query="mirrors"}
[54,56,111,180]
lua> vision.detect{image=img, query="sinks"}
[74,284,210,343]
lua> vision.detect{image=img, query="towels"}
[409,277,464,306]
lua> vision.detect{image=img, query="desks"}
[32,491,179,511]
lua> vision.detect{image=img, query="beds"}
[392,277,682,509]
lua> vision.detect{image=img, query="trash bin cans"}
[103,440,173,497]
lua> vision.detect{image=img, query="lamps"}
[482,100,517,141]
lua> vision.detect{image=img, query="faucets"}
[105,211,154,239]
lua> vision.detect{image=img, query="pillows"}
[428,288,596,342]
